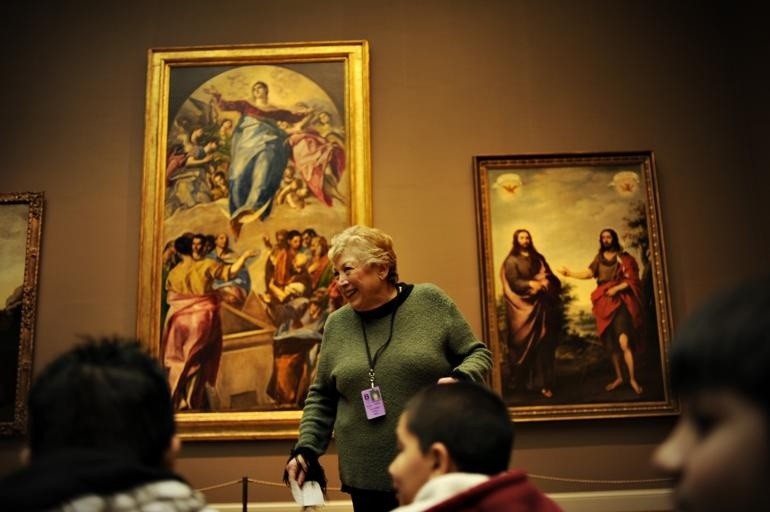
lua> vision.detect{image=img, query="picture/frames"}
[472,150,681,423]
[0,191,44,437]
[135,40,373,440]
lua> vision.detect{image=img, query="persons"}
[500,230,562,399]
[285,224,493,512]
[557,229,646,396]
[0,332,214,512]
[388,381,565,512]
[648,271,770,512]
